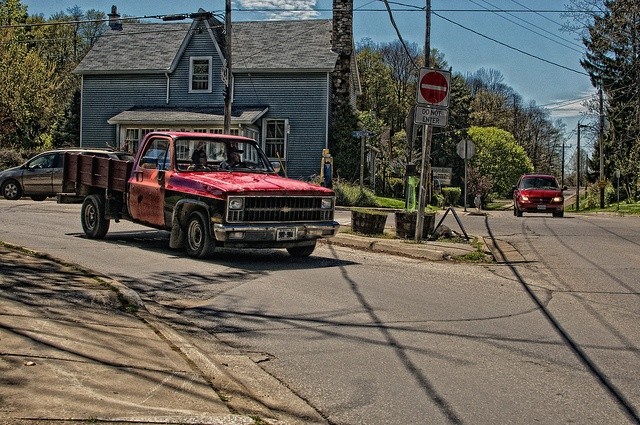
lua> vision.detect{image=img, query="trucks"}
[58,132,340,258]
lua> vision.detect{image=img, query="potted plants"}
[395,210,432,240]
[425,207,435,234]
[351,209,388,234]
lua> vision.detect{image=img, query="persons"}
[220,146,245,169]
[187,150,211,171]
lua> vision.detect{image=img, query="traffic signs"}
[414,105,448,127]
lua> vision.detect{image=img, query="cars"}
[1,146,135,201]
[513,173,566,218]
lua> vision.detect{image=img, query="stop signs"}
[416,68,451,109]
